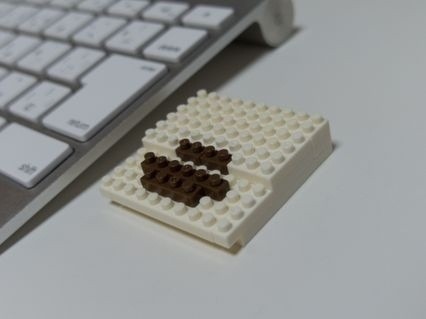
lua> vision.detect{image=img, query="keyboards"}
[1,0,295,247]
